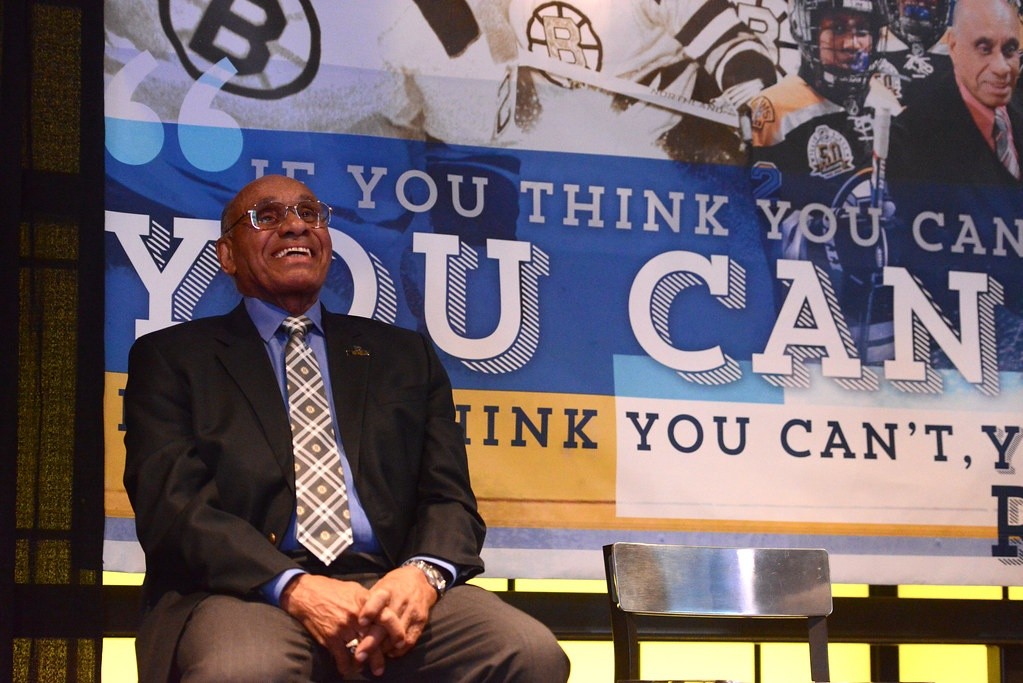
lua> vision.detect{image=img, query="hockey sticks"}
[517,45,742,130]
[857,102,893,365]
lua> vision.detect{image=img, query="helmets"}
[884,0,950,43]
[789,0,887,95]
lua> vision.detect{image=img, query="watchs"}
[400,561,446,600]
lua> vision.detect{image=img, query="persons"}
[105,0,1023,371]
[123,174,572,683]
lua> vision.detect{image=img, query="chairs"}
[603,542,833,683]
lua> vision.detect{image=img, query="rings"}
[345,638,359,648]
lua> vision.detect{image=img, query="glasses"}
[222,200,332,230]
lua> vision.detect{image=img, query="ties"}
[281,315,354,566]
[994,106,1021,181]
